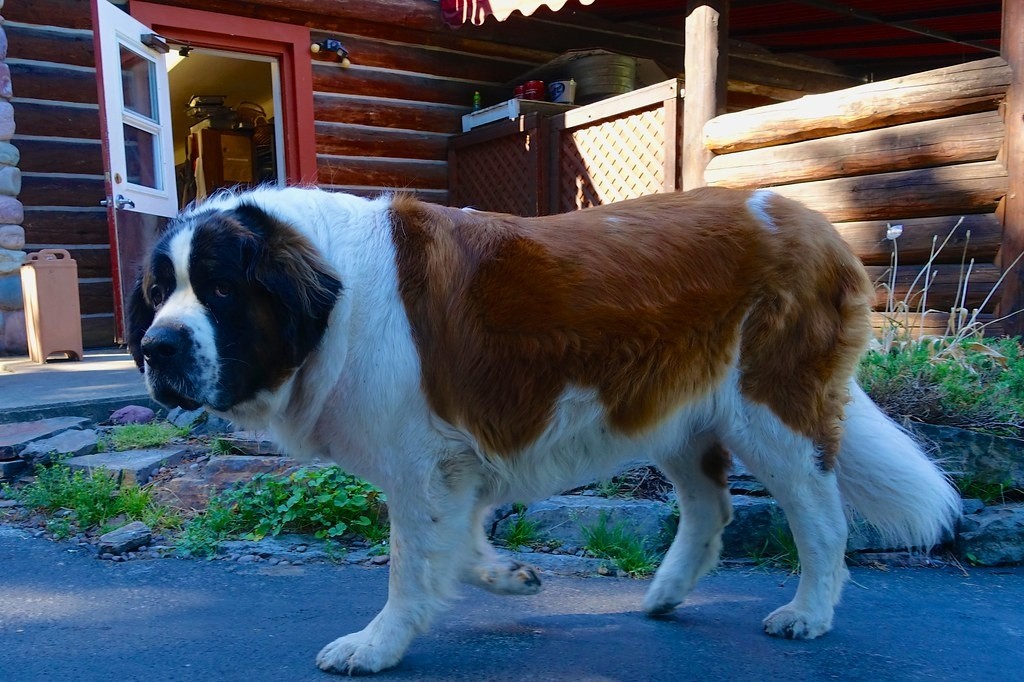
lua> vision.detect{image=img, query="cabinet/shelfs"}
[186,128,255,198]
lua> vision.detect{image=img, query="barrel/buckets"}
[548,78,577,104]
[513,86,524,100]
[523,80,545,101]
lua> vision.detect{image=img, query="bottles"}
[473,91,481,112]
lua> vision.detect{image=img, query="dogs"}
[124,159,964,677]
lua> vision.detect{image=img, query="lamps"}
[310,37,351,70]
